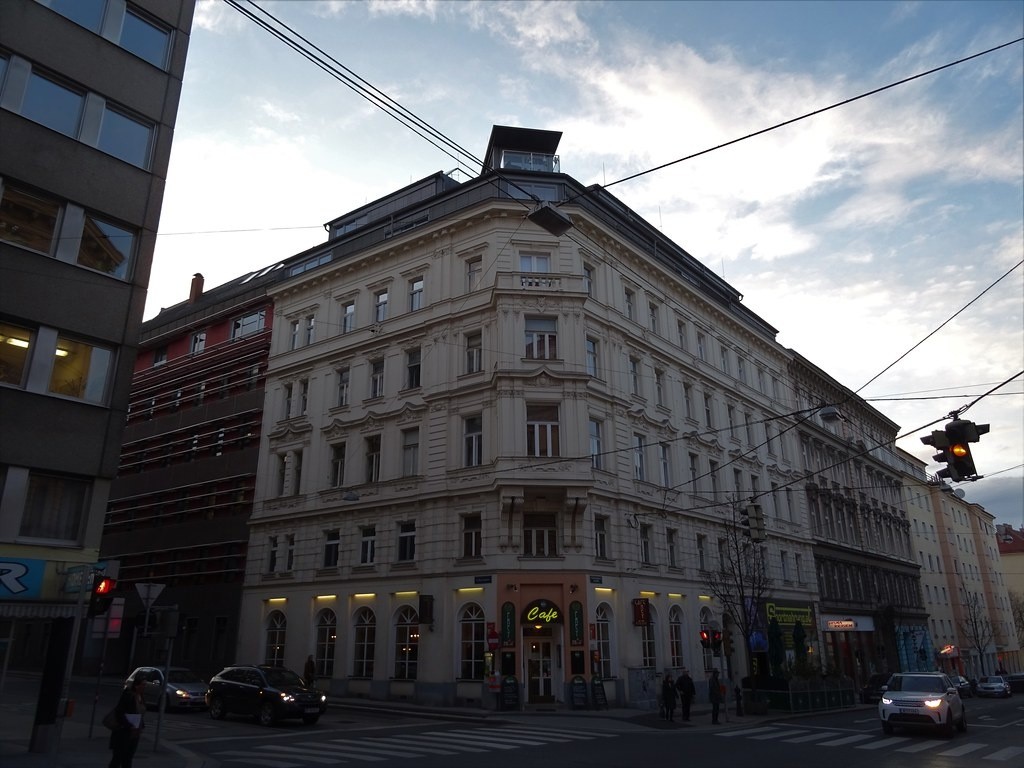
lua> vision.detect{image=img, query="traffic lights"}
[87,575,117,617]
[740,503,767,541]
[723,630,735,655]
[920,420,992,481]
[700,630,710,649]
[712,631,722,648]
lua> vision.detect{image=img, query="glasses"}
[683,670,689,673]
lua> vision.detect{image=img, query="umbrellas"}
[793,619,808,663]
[767,617,786,667]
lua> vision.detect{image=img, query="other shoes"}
[712,720,722,724]
[670,719,674,723]
[684,719,690,721]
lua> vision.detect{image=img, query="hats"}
[712,667,720,673]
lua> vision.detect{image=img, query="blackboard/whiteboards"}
[571,676,589,706]
[592,676,608,705]
[503,676,520,708]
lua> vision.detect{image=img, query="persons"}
[660,675,679,722]
[995,668,1007,676]
[108,672,147,768]
[708,671,722,724]
[304,655,314,687]
[676,669,696,721]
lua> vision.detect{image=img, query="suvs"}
[203,664,334,728]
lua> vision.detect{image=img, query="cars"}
[879,672,968,739]
[976,676,1012,698]
[949,675,974,698]
[123,666,212,713]
[1006,673,1024,690]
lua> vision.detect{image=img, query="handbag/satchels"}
[102,710,124,732]
[659,708,666,719]
